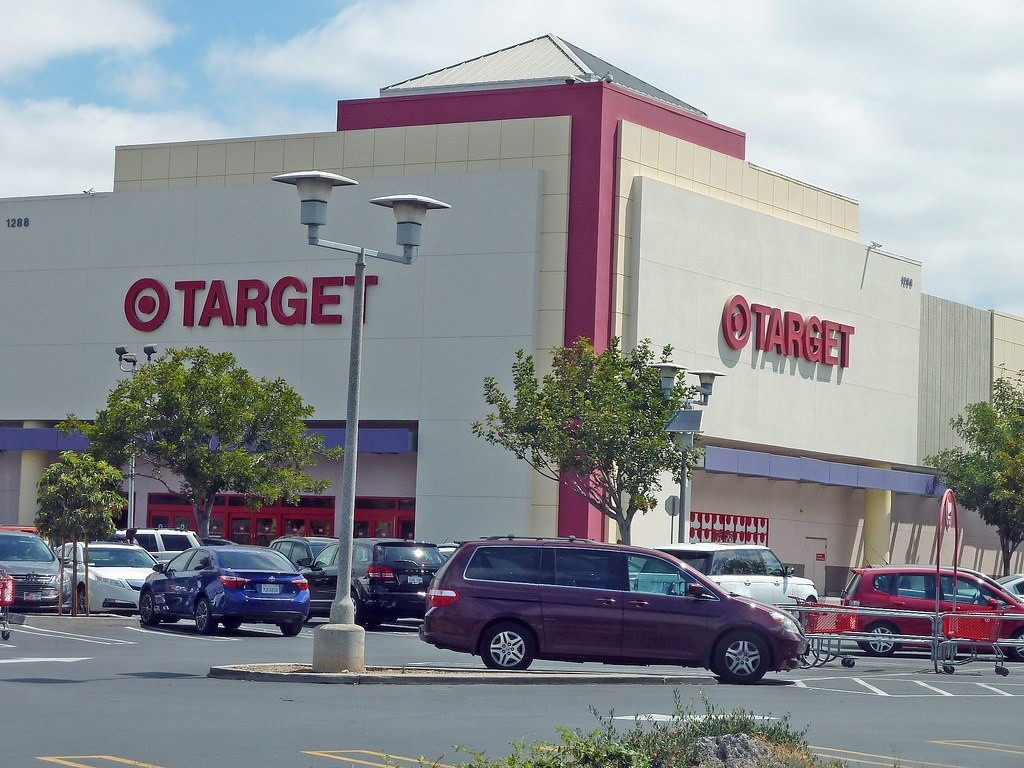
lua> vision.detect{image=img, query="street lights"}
[269,169,451,672]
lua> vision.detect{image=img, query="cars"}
[52,540,162,616]
[956,572,1024,607]
[0,523,76,615]
[139,545,310,638]
[437,541,460,564]
[268,535,340,574]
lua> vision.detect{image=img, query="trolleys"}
[931,605,1016,677]
[787,595,860,668]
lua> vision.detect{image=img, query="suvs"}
[632,541,819,626]
[298,537,447,629]
[1,524,247,572]
[417,533,809,687]
[836,564,1024,662]
